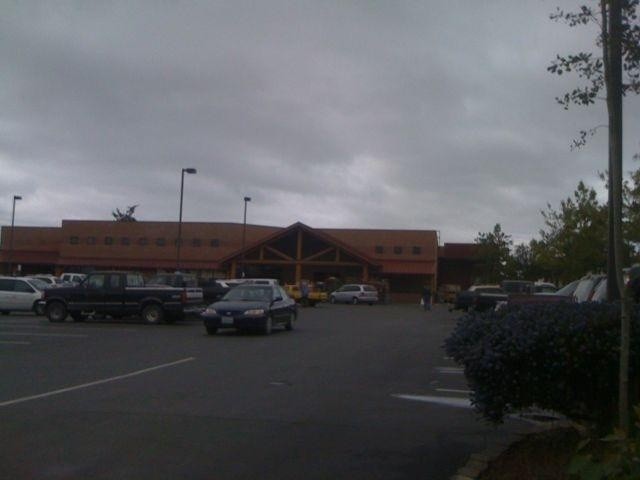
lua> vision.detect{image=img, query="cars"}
[467,262,639,311]
[0,271,298,334]
[330,283,378,305]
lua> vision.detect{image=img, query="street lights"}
[241,196,254,269]
[178,168,198,268]
[8,194,23,272]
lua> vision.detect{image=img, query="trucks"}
[281,280,328,307]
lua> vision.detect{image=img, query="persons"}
[422,285,432,311]
[301,282,308,307]
